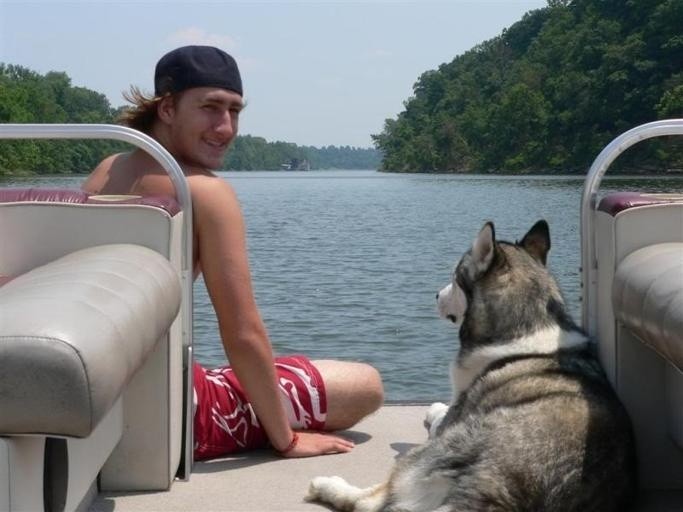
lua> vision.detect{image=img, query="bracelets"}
[276,433,299,454]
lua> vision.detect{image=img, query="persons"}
[80,45,383,466]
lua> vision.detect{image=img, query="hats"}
[154,45,243,98]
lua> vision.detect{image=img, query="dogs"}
[305,217,639,510]
